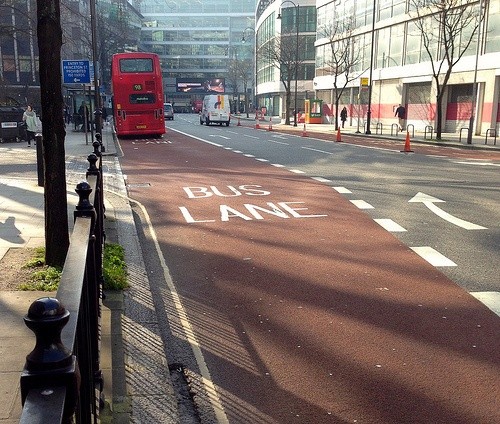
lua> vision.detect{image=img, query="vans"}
[199,95,231,126]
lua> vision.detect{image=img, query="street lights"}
[241,27,259,120]
[276,0,299,126]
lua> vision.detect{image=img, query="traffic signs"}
[62,60,90,84]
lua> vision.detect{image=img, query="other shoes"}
[34,142,36,145]
[28,144,30,147]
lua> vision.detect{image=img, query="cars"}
[163,103,174,120]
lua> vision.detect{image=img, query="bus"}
[111,52,165,139]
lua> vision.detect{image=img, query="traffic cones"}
[255,118,261,129]
[237,117,242,126]
[268,117,274,131]
[399,132,414,152]
[335,127,343,142]
[301,124,309,137]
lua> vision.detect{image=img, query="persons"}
[340,107,347,128]
[23,105,36,147]
[394,104,405,132]
[78,101,91,132]
[100,104,107,122]
